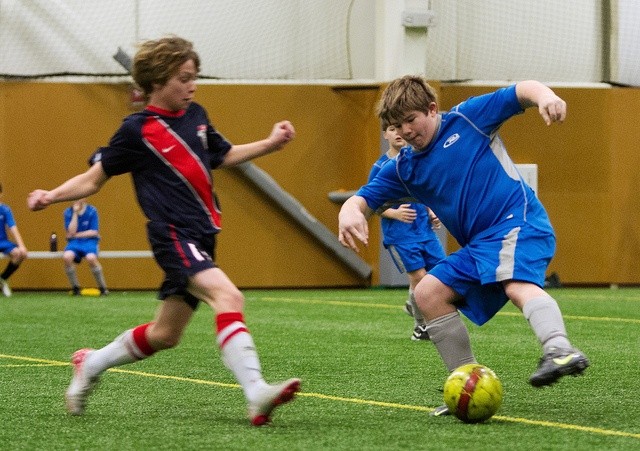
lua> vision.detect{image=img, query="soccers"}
[442,364,502,423]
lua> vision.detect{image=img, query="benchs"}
[0,250,152,298]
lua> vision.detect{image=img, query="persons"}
[337,73,590,418]
[27,36,300,426]
[64,196,109,296]
[367,116,448,344]
[0,183,27,297]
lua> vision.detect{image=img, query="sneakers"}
[529,348,591,388]
[66,348,99,416]
[248,378,301,425]
[411,325,428,341]
[0,278,12,297]
[429,405,450,415]
